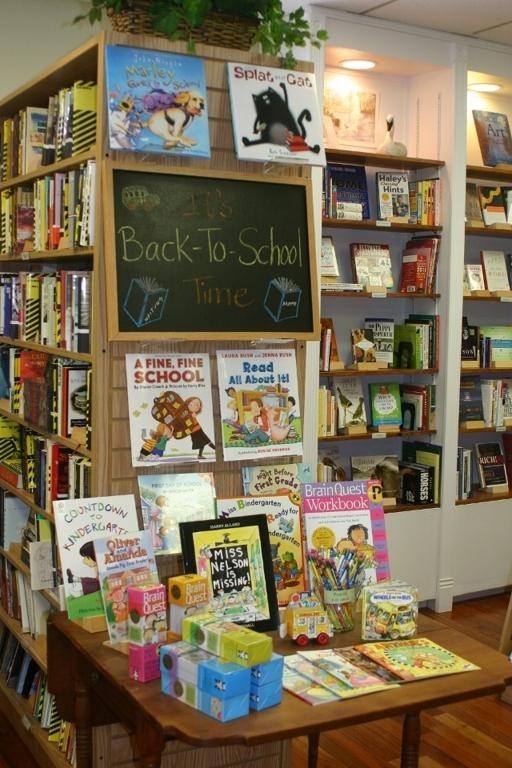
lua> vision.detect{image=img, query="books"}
[321,158,511,511]
[54,352,483,720]
[474,110,511,167]
[0,78,99,768]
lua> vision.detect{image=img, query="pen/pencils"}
[305,546,371,633]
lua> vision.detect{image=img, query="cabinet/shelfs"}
[449,164,512,613]
[320,141,449,614]
[0,28,320,768]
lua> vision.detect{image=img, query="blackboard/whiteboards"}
[101,159,321,341]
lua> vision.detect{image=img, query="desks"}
[43,590,512,768]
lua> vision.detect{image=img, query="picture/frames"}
[177,515,281,631]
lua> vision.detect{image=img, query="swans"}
[377,113,408,158]
